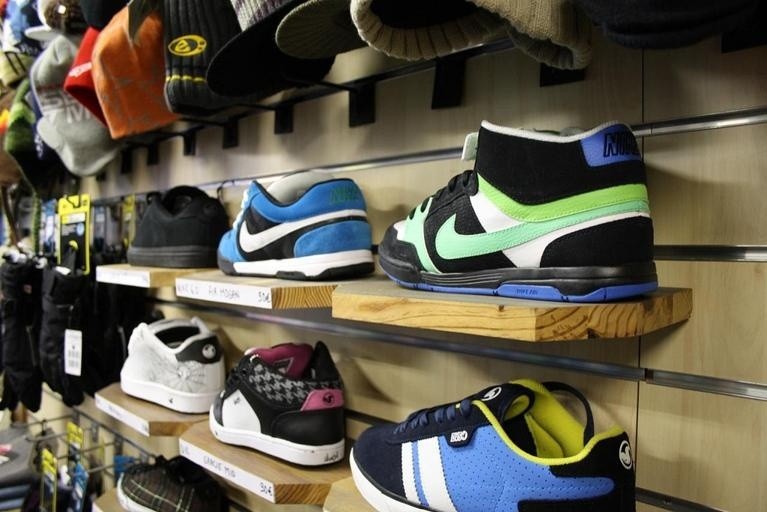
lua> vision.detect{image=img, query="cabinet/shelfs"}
[0,0,691,511]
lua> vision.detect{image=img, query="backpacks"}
[0,251,109,414]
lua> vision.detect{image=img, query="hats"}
[29,3,592,181]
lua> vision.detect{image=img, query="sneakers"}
[209,341,347,470]
[374,118,665,301]
[119,316,227,413]
[124,184,229,268]
[348,380,637,510]
[217,179,376,281]
[115,456,231,511]
